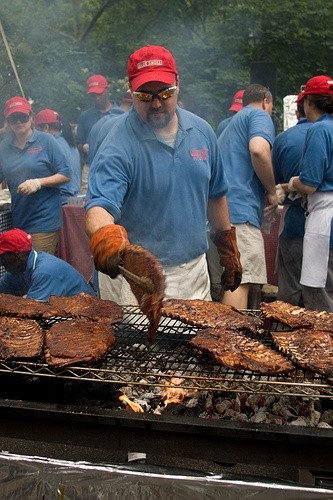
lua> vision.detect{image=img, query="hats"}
[294,75,333,103]
[0,228,32,254]
[127,45,178,91]
[85,74,108,95]
[228,89,245,112]
[3,96,33,118]
[31,109,61,124]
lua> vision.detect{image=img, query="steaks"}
[0,243,333,378]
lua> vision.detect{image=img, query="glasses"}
[7,116,29,124]
[129,75,178,102]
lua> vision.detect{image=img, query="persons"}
[84,45,244,333]
[34,109,82,206]
[0,228,96,301]
[0,96,70,256]
[216,89,246,139]
[274,75,333,314]
[75,75,112,150]
[271,90,315,329]
[206,84,278,310]
[83,90,134,164]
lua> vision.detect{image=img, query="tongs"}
[118,259,165,292]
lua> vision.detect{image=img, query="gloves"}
[89,224,130,279]
[16,178,41,196]
[210,226,242,292]
